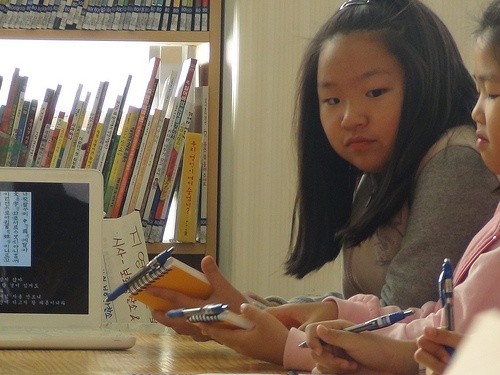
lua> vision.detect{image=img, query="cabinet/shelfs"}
[0,0,223,275]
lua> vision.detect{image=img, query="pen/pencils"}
[166,303,228,318]
[438,258,454,357]
[298,308,417,349]
[105,246,177,303]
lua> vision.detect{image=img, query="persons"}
[151,0,500,343]
[192,0,500,375]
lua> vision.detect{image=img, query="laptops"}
[0,167,137,350]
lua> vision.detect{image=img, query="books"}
[1,0,210,32]
[182,305,255,333]
[128,257,214,314]
[1,55,212,245]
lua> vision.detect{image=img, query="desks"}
[0,315,313,374]
